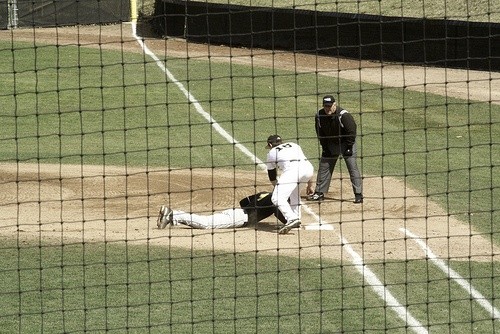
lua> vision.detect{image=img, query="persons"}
[156,188,300,229]
[306,95,363,203]
[267,135,314,234]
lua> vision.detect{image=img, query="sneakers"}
[354,193,363,203]
[157,205,173,230]
[306,192,324,201]
[290,224,301,231]
[278,218,301,234]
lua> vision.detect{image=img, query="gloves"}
[344,147,352,156]
[323,144,331,156]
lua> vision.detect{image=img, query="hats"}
[265,134,282,149]
[323,95,335,106]
